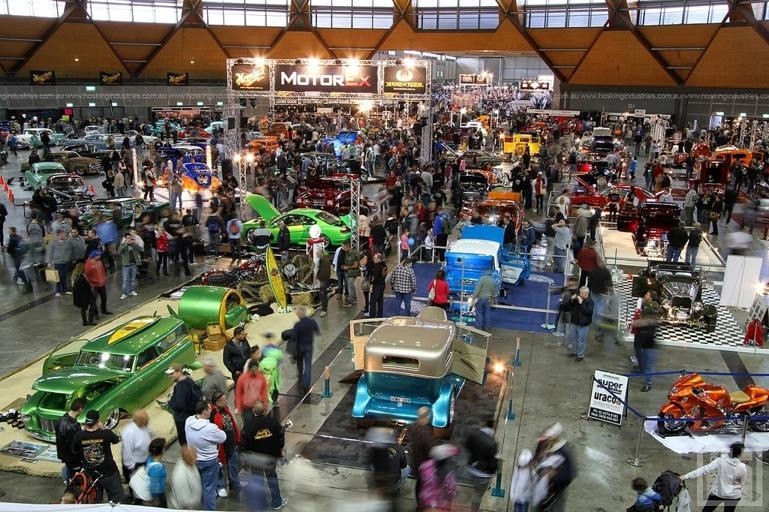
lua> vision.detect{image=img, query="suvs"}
[441,227,533,311]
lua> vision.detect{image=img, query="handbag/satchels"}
[427,285,436,301]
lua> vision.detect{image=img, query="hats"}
[82,410,100,425]
[89,250,103,258]
[406,406,569,477]
[163,362,181,375]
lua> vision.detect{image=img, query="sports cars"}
[242,192,353,248]
[630,257,719,333]
[616,201,686,257]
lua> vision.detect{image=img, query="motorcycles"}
[655,369,769,436]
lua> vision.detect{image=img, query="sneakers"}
[238,481,249,488]
[217,487,228,498]
[120,293,127,300]
[640,384,651,393]
[310,291,380,321]
[55,290,114,327]
[129,291,139,296]
[567,353,585,362]
[155,253,197,281]
[271,496,290,510]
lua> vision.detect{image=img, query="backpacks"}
[129,463,165,502]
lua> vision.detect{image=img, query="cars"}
[15,315,193,444]
[347,304,491,427]
